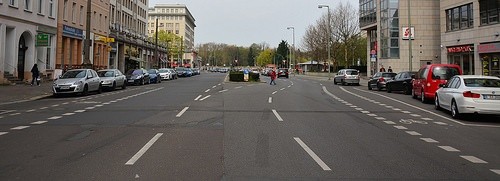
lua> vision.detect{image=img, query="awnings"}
[160,57,169,62]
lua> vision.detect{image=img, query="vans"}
[411,63,463,103]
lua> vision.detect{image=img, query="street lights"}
[318,5,331,74]
[287,26,295,71]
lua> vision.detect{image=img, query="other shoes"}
[31,84,32,85]
[38,85,40,86]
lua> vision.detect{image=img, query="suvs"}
[334,68,361,86]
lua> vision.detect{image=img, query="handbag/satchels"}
[37,75,41,80]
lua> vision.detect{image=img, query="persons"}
[270,69,277,85]
[31,64,40,87]
[380,64,392,73]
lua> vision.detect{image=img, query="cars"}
[200,65,279,76]
[277,68,288,78]
[97,68,128,92]
[386,71,419,95]
[147,69,162,84]
[434,74,500,119]
[158,68,172,81]
[170,67,200,80]
[368,71,398,91]
[123,68,151,85]
[52,68,103,98]
